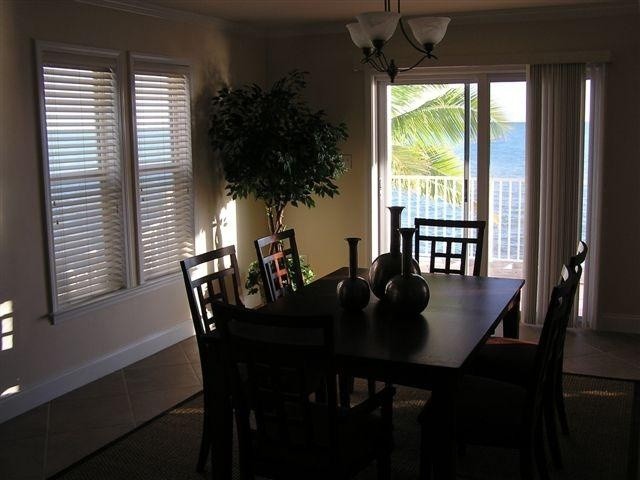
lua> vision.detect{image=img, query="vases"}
[336,237,370,312]
[369,207,422,301]
[383,228,430,316]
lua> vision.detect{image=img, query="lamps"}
[346,0,451,83]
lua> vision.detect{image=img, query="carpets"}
[47,367,639,479]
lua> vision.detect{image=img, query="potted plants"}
[206,71,351,306]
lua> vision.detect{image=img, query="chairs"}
[254,228,376,405]
[416,265,577,479]
[432,240,588,434]
[415,218,487,275]
[180,246,326,471]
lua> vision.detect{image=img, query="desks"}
[200,266,525,479]
